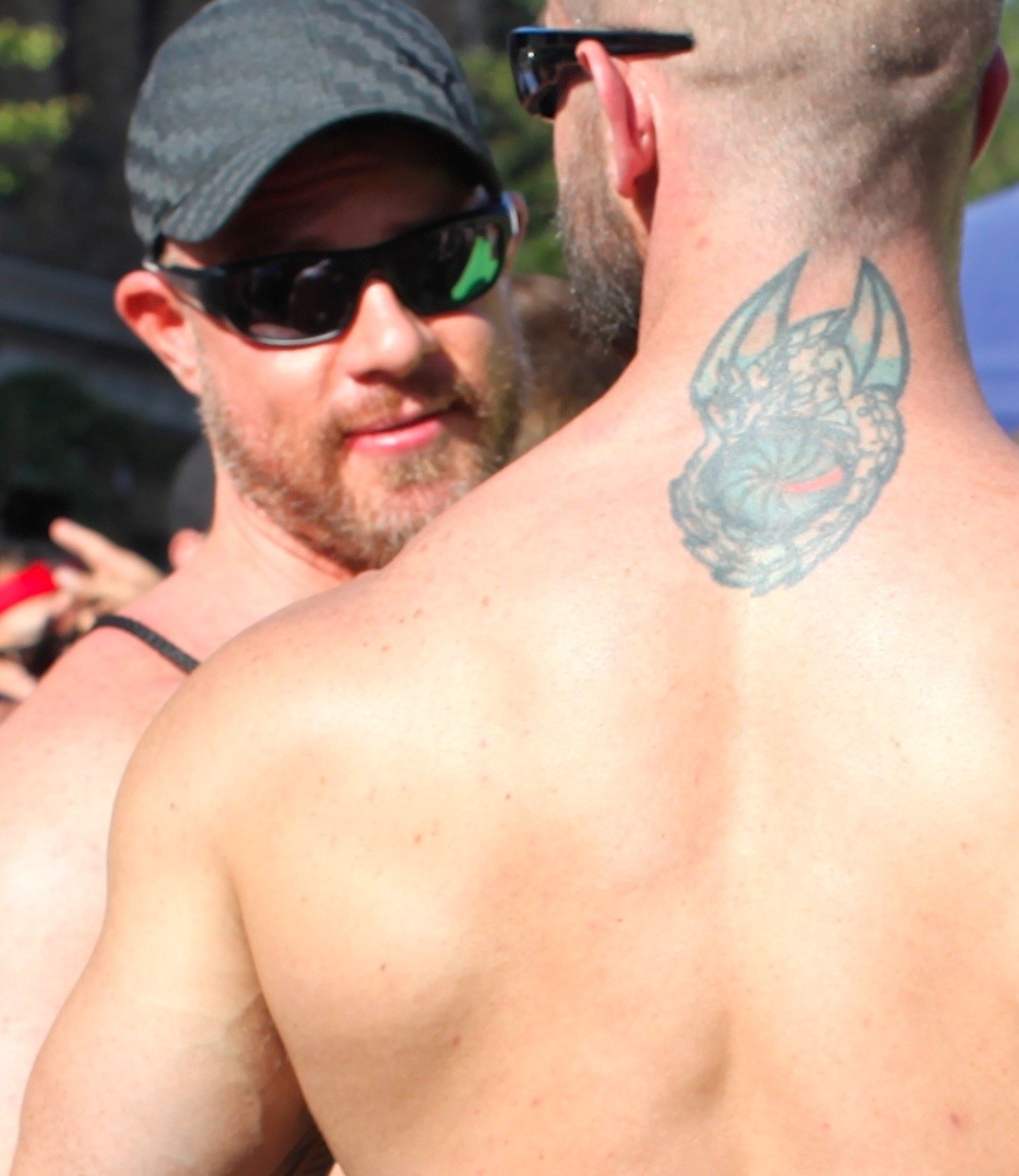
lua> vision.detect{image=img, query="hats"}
[126,0,500,251]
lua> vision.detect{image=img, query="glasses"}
[512,27,694,122]
[143,184,520,348]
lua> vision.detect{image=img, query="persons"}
[0,0,639,1176]
[17,0,1018,1176]
